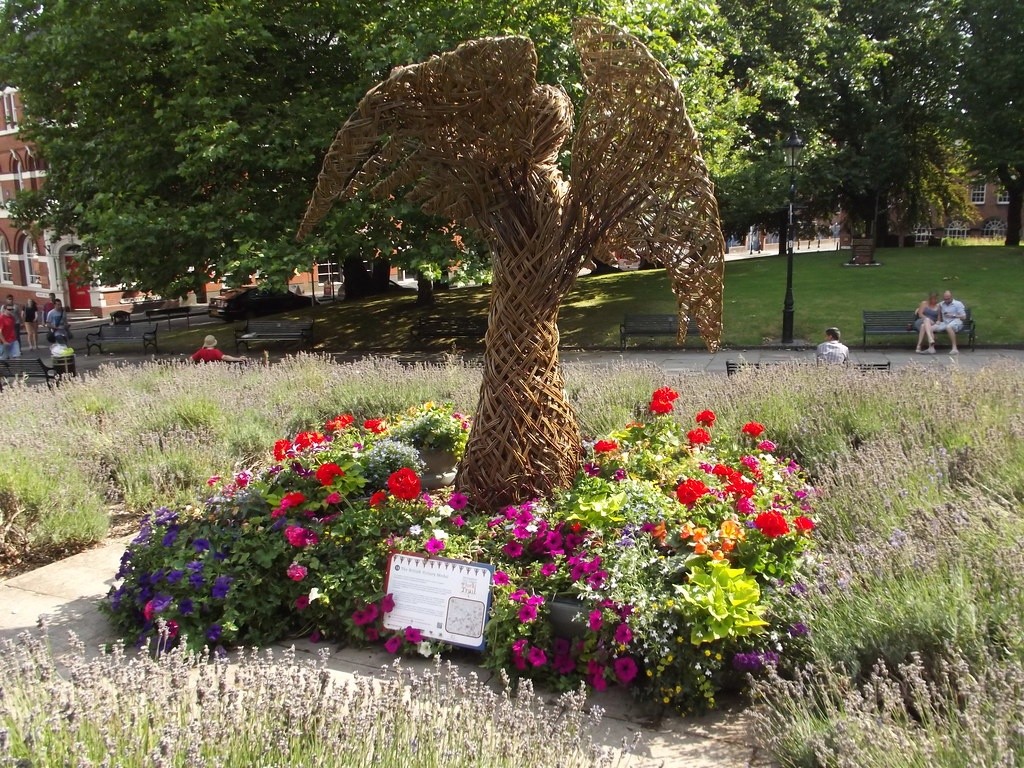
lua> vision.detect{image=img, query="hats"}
[204,334,217,347]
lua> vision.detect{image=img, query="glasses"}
[8,298,12,300]
[7,309,14,311]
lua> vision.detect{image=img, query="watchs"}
[953,313,955,317]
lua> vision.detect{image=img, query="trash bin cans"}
[53,353,76,380]
[110,310,132,326]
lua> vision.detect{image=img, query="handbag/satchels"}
[46,329,56,343]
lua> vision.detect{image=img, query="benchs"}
[409,315,488,349]
[863,309,976,353]
[0,358,62,390]
[726,361,891,377]
[86,323,158,356]
[234,318,315,354]
[620,314,701,354]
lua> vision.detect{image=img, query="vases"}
[415,449,458,488]
[547,591,589,635]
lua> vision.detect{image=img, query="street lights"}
[778,129,806,343]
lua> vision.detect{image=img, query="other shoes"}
[922,349,937,355]
[930,339,936,344]
[916,346,922,354]
[949,350,959,355]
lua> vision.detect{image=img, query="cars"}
[207,285,320,323]
[337,269,418,304]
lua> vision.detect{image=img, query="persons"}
[816,327,850,365]
[188,335,247,363]
[913,290,966,354]
[0,293,71,368]
